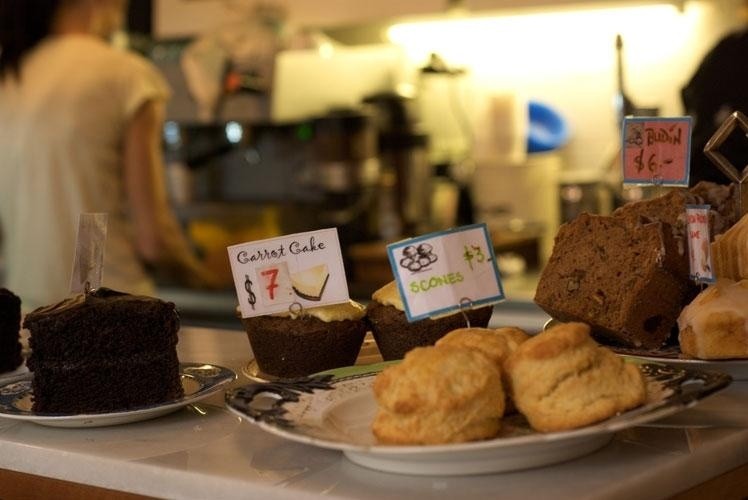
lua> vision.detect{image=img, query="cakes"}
[432,327,530,416]
[366,346,503,442]
[503,323,648,432]
[235,299,368,377]
[22,286,181,417]
[367,276,493,360]
[0,287,24,373]
[533,178,748,359]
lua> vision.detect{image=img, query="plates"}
[538,315,747,372]
[0,360,238,430]
[225,350,732,477]
[241,329,380,385]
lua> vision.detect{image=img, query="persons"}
[0,0,216,366]
